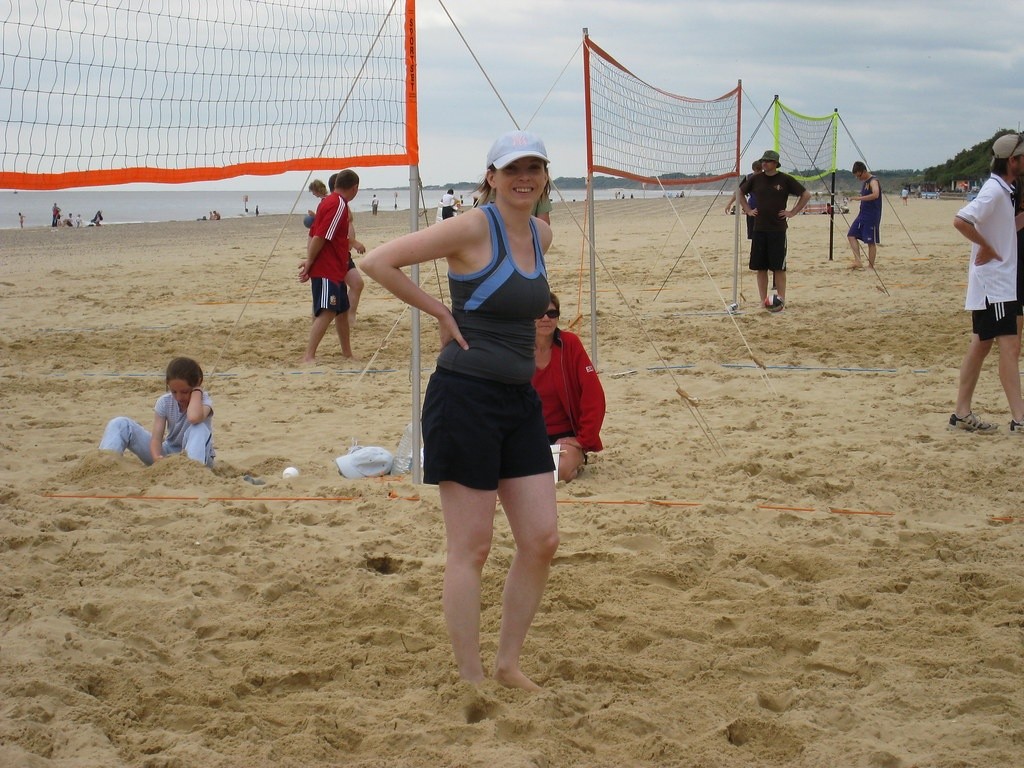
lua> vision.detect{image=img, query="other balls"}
[283,467,299,479]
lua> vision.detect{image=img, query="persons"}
[842,197,849,214]
[534,291,606,484]
[19,213,25,228]
[52,203,62,227]
[615,192,633,199]
[209,211,220,220]
[676,191,685,197]
[360,130,559,691]
[948,134,1024,433]
[76,214,82,228]
[902,188,909,206]
[822,203,832,214]
[442,188,458,220]
[98,357,217,466]
[372,194,379,215]
[815,192,821,200]
[92,210,103,223]
[847,162,882,269]
[62,213,74,227]
[256,205,259,216]
[725,150,811,308]
[299,169,366,363]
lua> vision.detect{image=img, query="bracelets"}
[190,389,202,393]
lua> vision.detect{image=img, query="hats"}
[337,447,391,478]
[991,134,1024,158]
[758,150,781,168]
[487,132,551,169]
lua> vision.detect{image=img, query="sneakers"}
[1010,418,1024,431]
[948,407,998,432]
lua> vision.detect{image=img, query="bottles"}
[391,423,414,475]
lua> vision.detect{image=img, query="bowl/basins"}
[283,467,298,479]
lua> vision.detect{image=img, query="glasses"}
[537,309,562,319]
[858,171,863,177]
[1010,135,1024,157]
